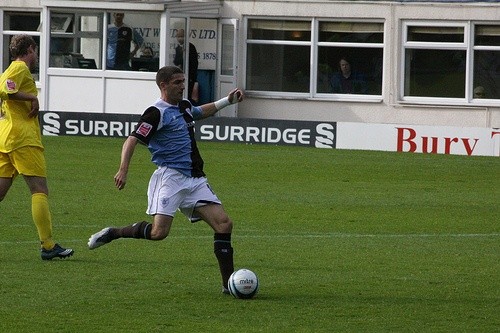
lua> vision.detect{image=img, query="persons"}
[88,65,245,295]
[173,29,199,106]
[106,12,153,72]
[0,33,74,262]
[474,86,486,98]
[329,56,368,94]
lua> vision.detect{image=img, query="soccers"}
[227,269,260,297]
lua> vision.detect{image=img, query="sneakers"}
[40,244,74,261]
[222,287,230,293]
[87,227,112,251]
